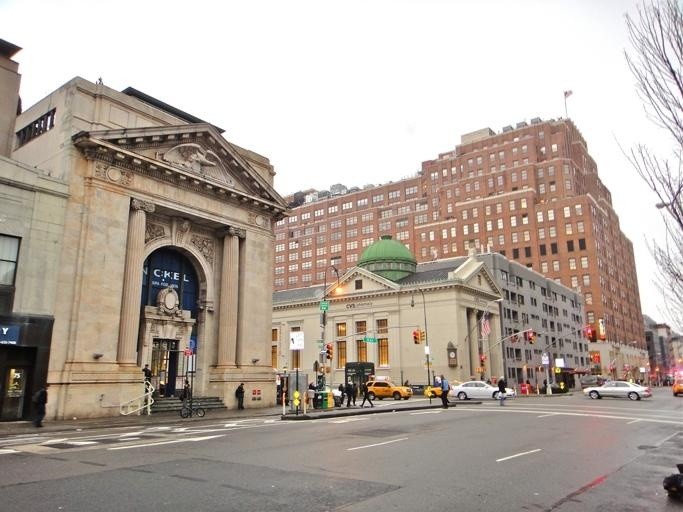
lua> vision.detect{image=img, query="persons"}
[32,383,50,427]
[498,376,506,406]
[142,364,152,404]
[309,382,316,390]
[180,380,194,417]
[238,383,245,410]
[339,382,373,408]
[543,377,547,394]
[440,375,450,409]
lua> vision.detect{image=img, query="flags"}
[565,90,573,98]
[481,311,491,337]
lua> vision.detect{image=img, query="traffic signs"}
[363,337,376,344]
[319,301,329,311]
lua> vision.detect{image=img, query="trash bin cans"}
[318,391,328,409]
[312,392,323,409]
[328,392,333,407]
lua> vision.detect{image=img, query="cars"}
[452,381,513,401]
[582,380,651,401]
[423,386,450,398]
[671,379,682,396]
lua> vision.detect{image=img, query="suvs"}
[362,381,412,400]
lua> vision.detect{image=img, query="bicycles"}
[179,398,205,418]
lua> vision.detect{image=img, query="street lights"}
[318,265,342,393]
[411,288,430,386]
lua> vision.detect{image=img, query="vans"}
[580,375,603,389]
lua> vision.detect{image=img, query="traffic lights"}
[587,329,592,339]
[412,330,424,344]
[326,345,332,360]
[528,336,534,345]
[479,355,487,367]
